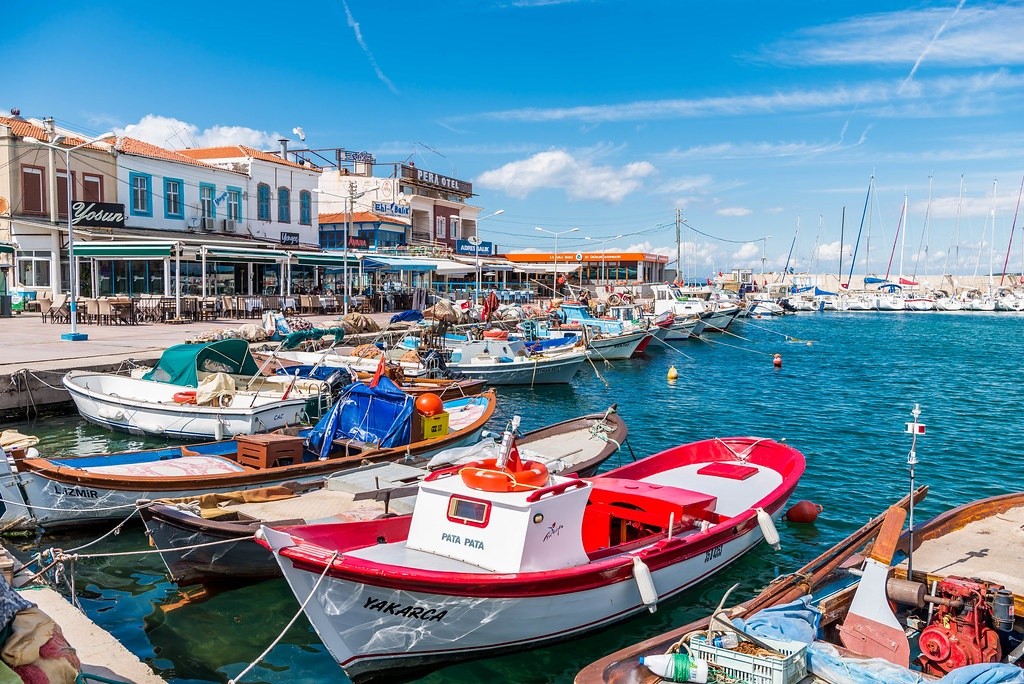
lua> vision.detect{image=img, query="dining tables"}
[65,300,86,323]
[108,301,130,325]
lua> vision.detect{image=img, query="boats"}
[9,387,498,526]
[61,339,308,438]
[136,403,627,584]
[124,283,785,422]
[573,484,1024,683]
[255,436,808,684]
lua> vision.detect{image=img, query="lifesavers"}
[173,391,198,404]
[607,293,621,306]
[462,459,550,493]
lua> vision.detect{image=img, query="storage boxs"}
[690,629,808,684]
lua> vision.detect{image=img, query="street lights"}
[584,235,622,285]
[311,186,381,316]
[450,210,504,308]
[534,227,580,301]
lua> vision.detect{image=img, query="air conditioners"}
[222,219,237,232]
[201,218,216,231]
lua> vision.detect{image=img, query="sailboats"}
[776,167,1024,312]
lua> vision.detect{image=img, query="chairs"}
[221,294,370,320]
[38,298,218,326]
[394,290,534,311]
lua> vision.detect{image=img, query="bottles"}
[639,654,708,683]
[704,634,738,649]
